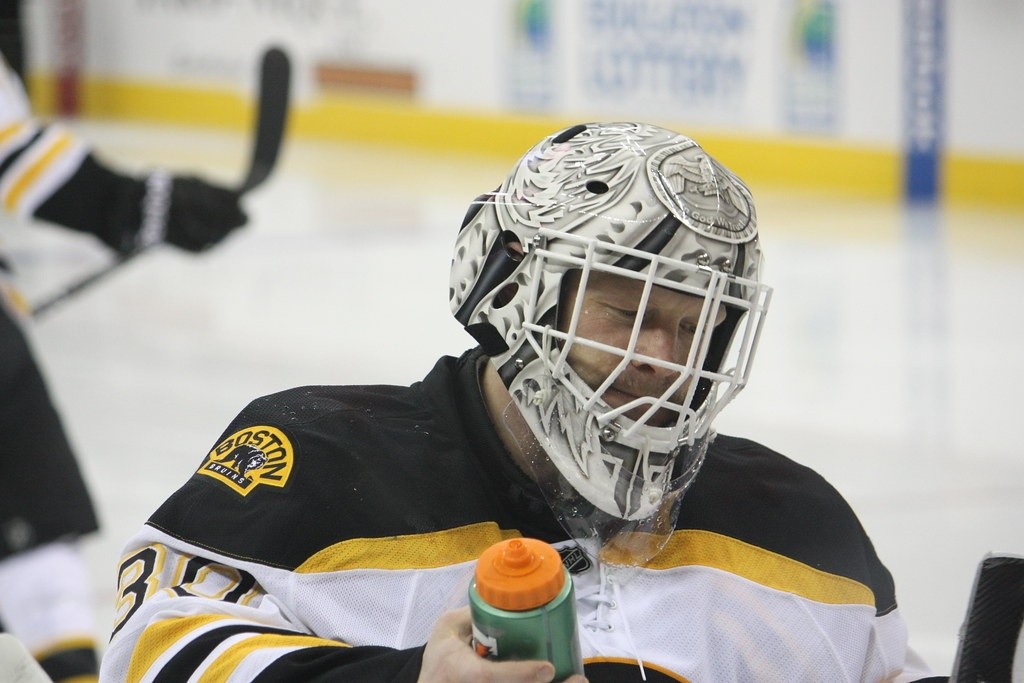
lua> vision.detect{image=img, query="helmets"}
[448,124,774,523]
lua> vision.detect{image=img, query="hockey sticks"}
[29,47,290,318]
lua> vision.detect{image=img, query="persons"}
[97,124,950,683]
[1,44,248,682]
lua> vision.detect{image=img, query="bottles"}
[467,538,583,682]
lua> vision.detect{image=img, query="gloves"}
[135,173,246,252]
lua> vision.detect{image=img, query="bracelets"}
[137,163,173,249]
